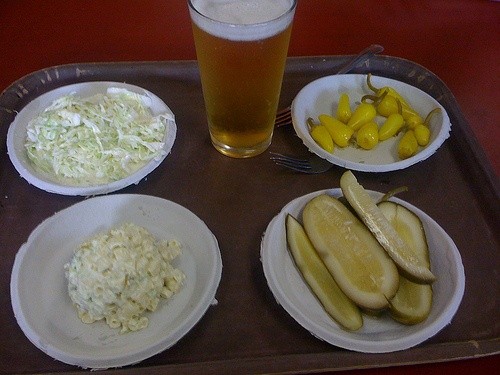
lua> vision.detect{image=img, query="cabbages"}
[25,87,176,184]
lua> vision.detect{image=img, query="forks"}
[269,151,333,174]
[275,44,385,128]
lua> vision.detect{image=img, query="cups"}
[188,0,297,159]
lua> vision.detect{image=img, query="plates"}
[6,82,177,195]
[291,74,451,173]
[10,194,223,368]
[260,188,466,354]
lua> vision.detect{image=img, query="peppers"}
[307,73,441,160]
